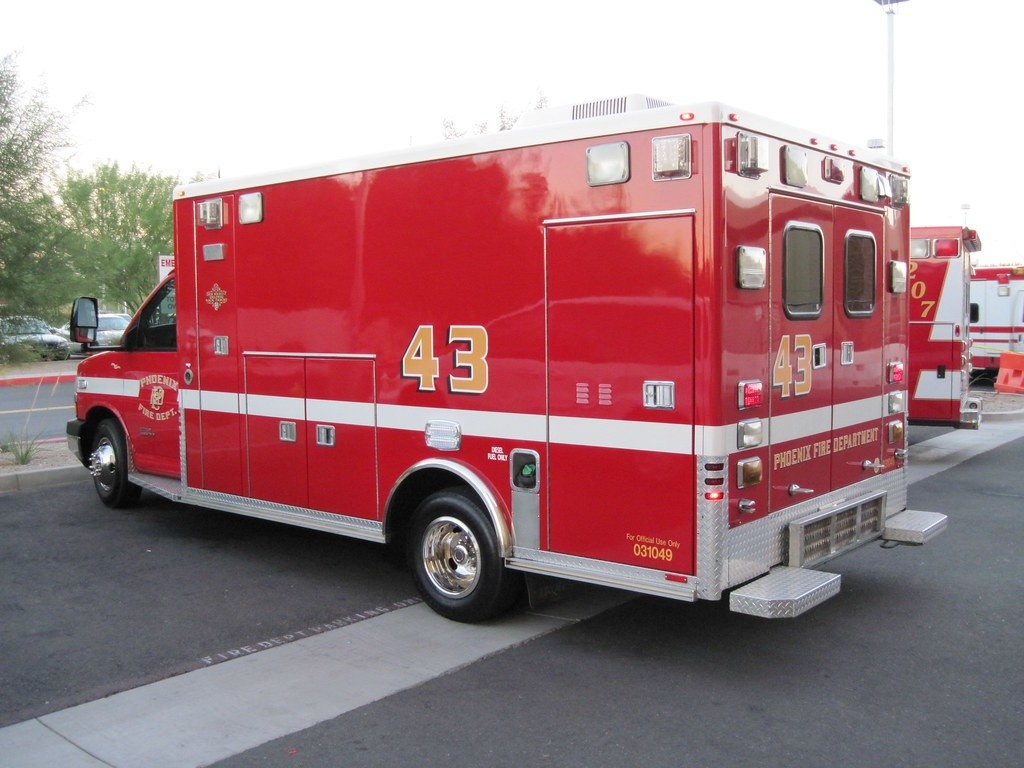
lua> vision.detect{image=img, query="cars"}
[48,314,130,359]
[111,312,132,323]
[0,315,72,361]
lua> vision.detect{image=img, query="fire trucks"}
[65,95,950,627]
[910,224,987,433]
[968,264,1023,384]
